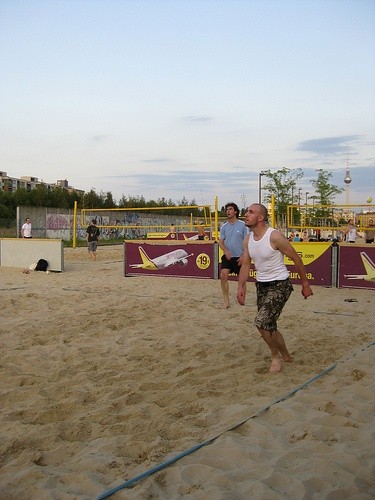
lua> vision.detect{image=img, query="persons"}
[21,218,32,238]
[220,203,248,309]
[237,203,314,373]
[289,231,307,242]
[364,220,375,244]
[341,219,362,243]
[87,220,100,259]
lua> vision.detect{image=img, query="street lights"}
[305,192,310,205]
[258,172,267,205]
[298,188,302,205]
[291,182,297,203]
[311,196,317,211]
